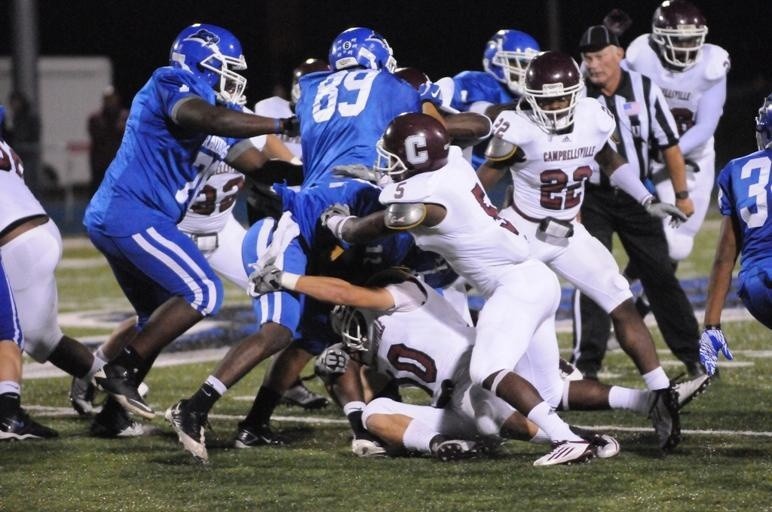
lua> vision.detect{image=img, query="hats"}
[578,25,622,52]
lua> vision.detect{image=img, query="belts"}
[587,176,648,196]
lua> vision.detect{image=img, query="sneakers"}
[577,429,619,459]
[650,389,677,451]
[351,438,394,459]
[232,423,291,448]
[0,411,57,441]
[670,374,709,412]
[71,378,90,403]
[278,384,328,409]
[94,412,144,437]
[72,398,105,415]
[432,434,488,461]
[92,363,155,420]
[533,441,594,468]
[165,399,210,465]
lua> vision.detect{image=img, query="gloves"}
[253,266,281,294]
[331,164,379,185]
[320,204,349,226]
[699,326,732,376]
[316,344,349,375]
[645,200,687,228]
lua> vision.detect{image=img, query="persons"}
[162,187,343,462]
[0,140,121,441]
[71,24,299,437]
[626,1,730,326]
[253,251,622,460]
[283,24,422,256]
[573,24,723,388]
[4,90,43,198]
[698,95,772,380]
[452,27,542,212]
[474,52,689,458]
[375,112,679,467]
[86,85,130,197]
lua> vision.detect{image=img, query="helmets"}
[652,0,707,67]
[292,59,330,88]
[374,112,448,188]
[483,29,538,96]
[525,51,583,131]
[331,300,370,352]
[170,23,248,107]
[329,28,396,75]
[756,94,772,150]
